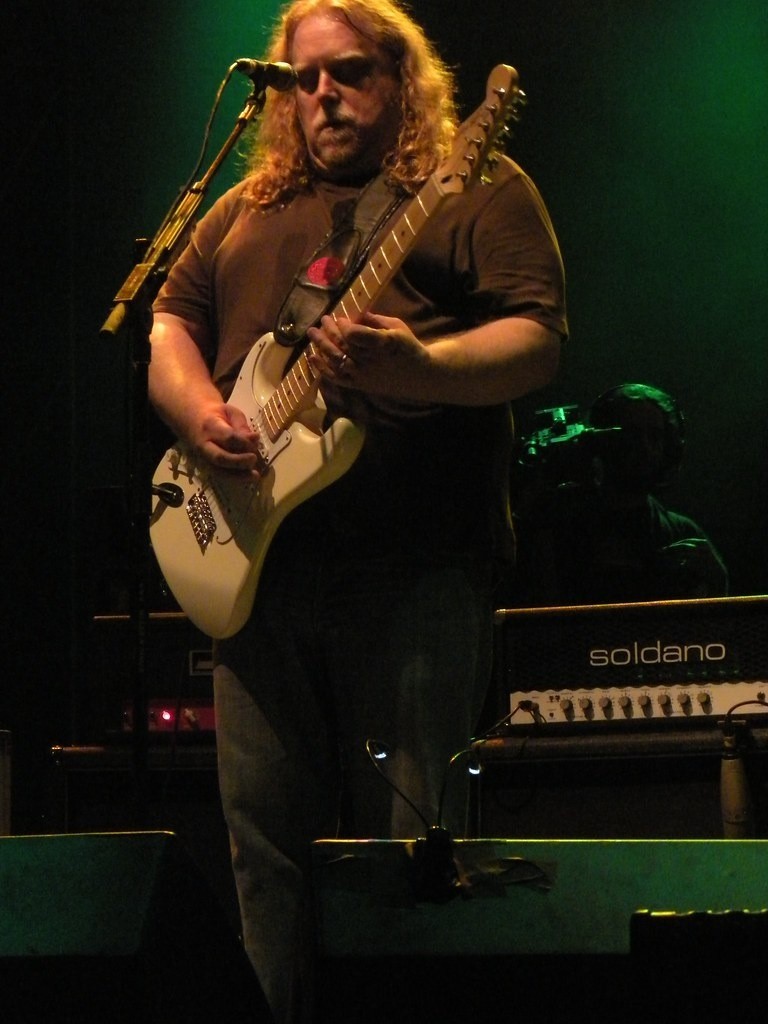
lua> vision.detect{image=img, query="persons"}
[146,0,572,1024]
[529,383,730,608]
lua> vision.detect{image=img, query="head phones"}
[585,380,691,478]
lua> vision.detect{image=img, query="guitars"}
[144,64,532,644]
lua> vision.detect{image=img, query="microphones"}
[236,58,299,92]
[720,717,755,839]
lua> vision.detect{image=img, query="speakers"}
[470,727,768,841]
[288,837,768,1024]
[0,830,274,1024]
[53,744,244,944]
